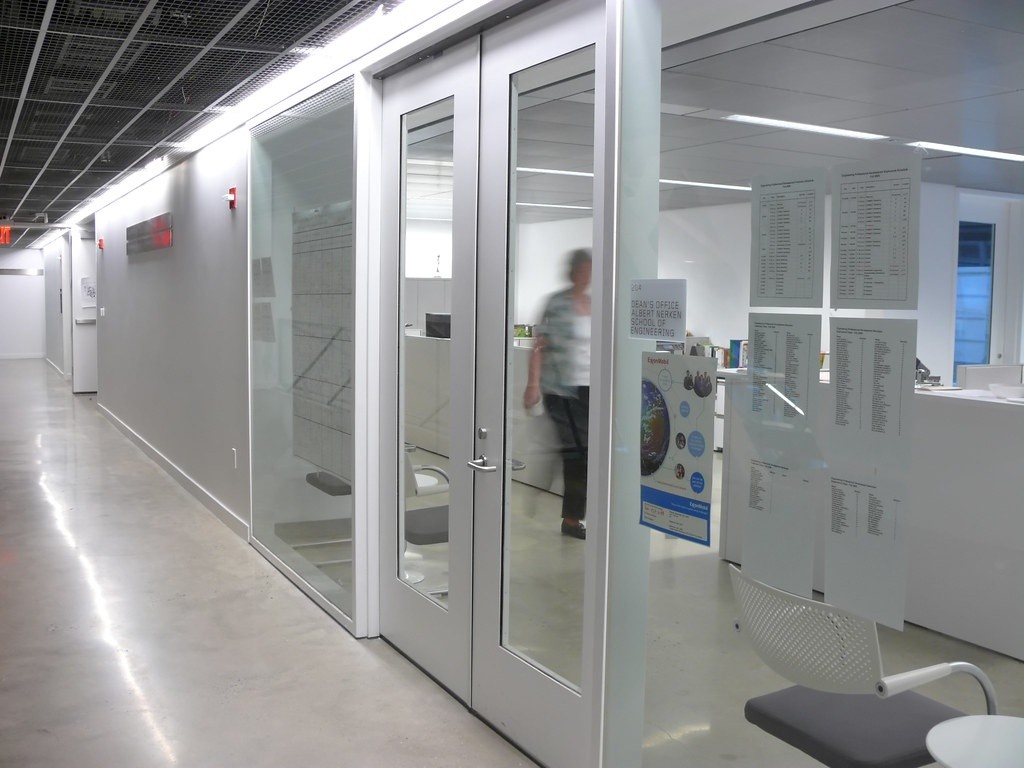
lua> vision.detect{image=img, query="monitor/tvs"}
[425,313,450,339]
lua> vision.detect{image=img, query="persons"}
[522,249,594,539]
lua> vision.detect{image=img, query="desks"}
[925,715,1024,768]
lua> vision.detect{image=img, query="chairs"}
[290,443,449,596]
[729,564,997,768]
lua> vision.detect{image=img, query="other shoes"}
[561,518,586,539]
